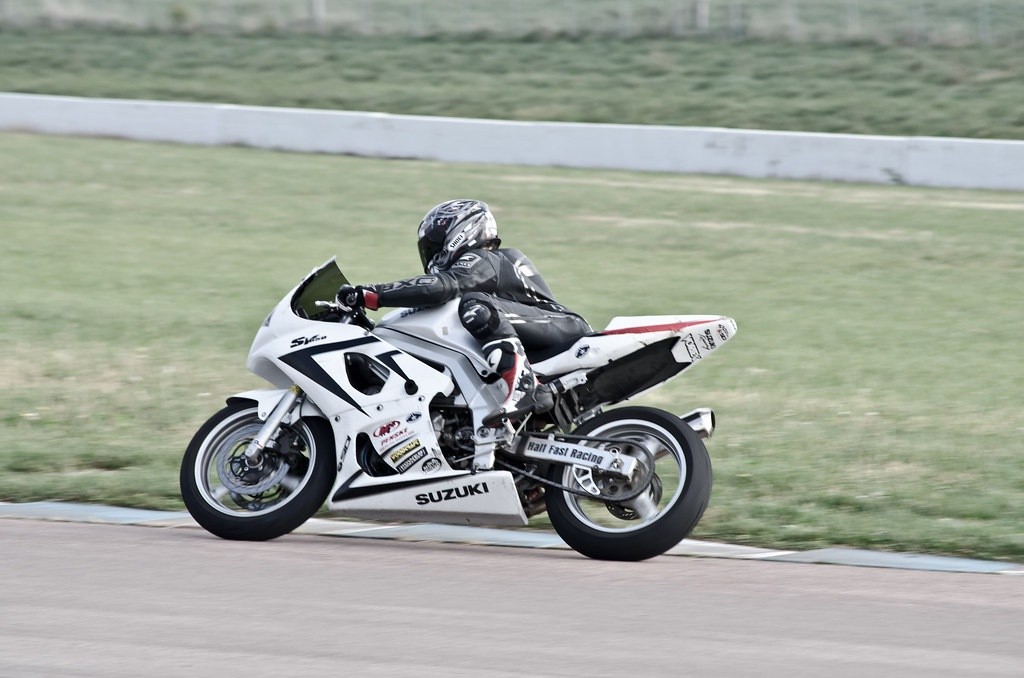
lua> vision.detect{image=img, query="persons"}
[335,199,593,428]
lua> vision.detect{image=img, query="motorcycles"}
[179,255,738,563]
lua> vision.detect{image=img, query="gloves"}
[335,284,379,311]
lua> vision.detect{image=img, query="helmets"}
[417,199,501,274]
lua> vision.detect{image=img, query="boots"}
[482,337,554,426]
